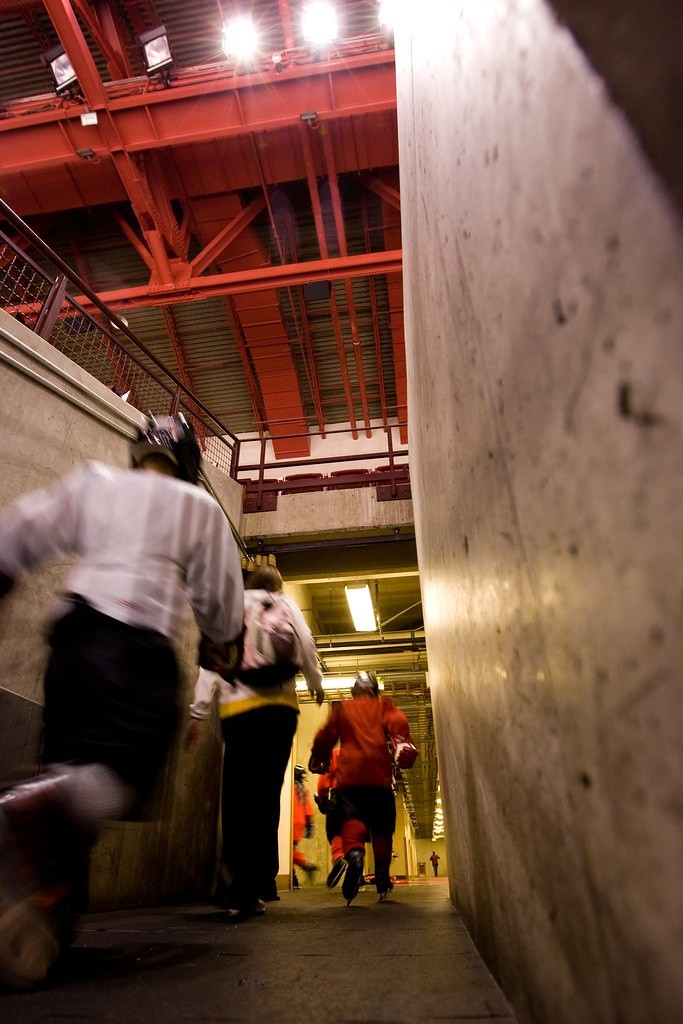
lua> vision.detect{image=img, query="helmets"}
[126,410,200,485]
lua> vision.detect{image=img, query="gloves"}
[0,572,13,599]
[394,742,421,769]
[309,750,331,775]
[196,624,247,687]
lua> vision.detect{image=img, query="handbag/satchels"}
[236,596,300,692]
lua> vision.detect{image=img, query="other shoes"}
[375,864,393,893]
[293,874,299,887]
[327,857,348,888]
[0,778,88,989]
[342,851,365,900]
[262,896,280,903]
[302,863,317,872]
[225,896,265,913]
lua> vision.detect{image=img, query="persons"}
[184,564,326,917]
[292,670,419,907]
[430,851,441,877]
[1,418,245,982]
